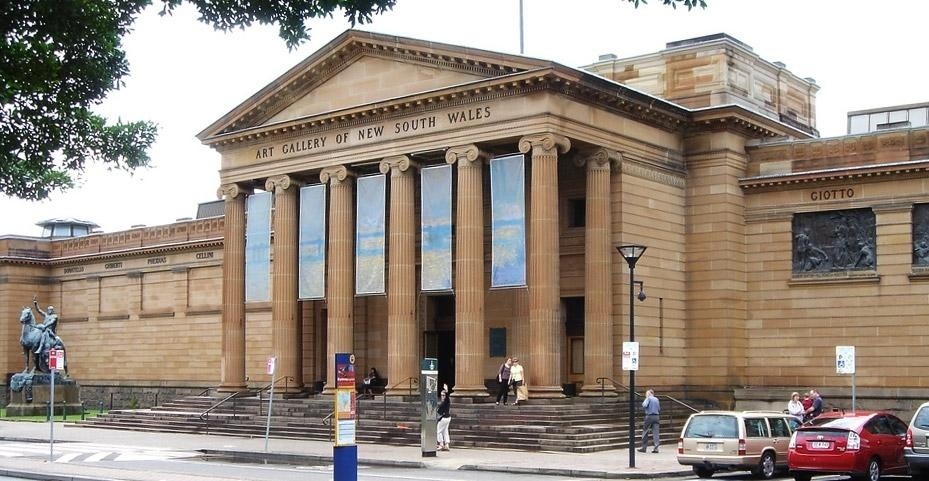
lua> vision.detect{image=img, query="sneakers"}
[651,450,658,453]
[496,401,519,406]
[437,446,449,451]
[637,449,645,452]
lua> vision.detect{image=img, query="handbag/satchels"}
[496,373,502,383]
[517,385,528,400]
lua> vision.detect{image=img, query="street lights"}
[616,244,647,470]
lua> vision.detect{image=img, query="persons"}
[852,242,874,267]
[637,389,661,453]
[788,392,805,430]
[510,357,525,406]
[805,243,829,271]
[793,228,811,272]
[802,393,812,411]
[33,298,58,354]
[803,389,823,423]
[436,384,451,451]
[364,368,378,395]
[496,356,512,405]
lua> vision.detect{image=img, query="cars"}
[902,401,929,481]
[786,409,910,481]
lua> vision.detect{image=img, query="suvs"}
[676,409,803,480]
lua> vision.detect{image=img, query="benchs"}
[362,379,387,392]
[484,379,501,395]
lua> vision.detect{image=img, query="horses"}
[17,306,44,375]
